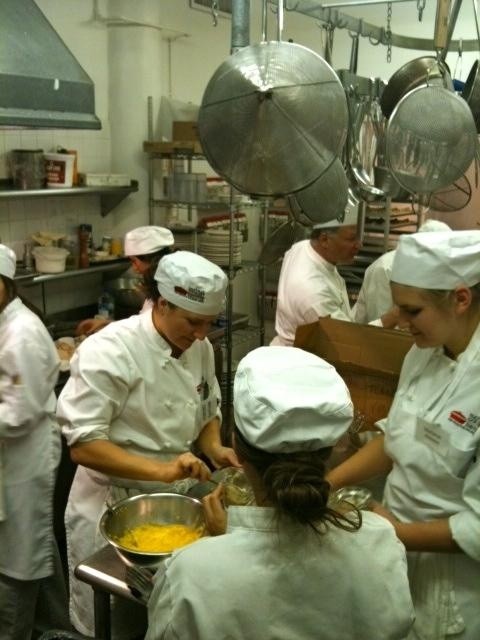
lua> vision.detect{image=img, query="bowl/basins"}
[103,275,148,306]
[98,494,210,565]
[31,248,69,273]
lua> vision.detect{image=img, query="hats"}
[312,197,358,230]
[153,251,228,316]
[390,220,480,291]
[232,344,355,454]
[124,225,175,257]
[0,244,17,280]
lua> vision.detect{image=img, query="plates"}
[163,225,244,271]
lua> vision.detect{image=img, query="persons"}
[0,238,65,640]
[73,225,179,338]
[58,246,244,638]
[322,227,480,640]
[348,216,454,328]
[265,183,399,349]
[143,342,416,638]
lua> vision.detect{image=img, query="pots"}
[382,0,480,141]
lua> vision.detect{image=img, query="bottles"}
[22,241,34,268]
[79,222,92,269]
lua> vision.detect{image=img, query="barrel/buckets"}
[95,280,116,318]
[8,148,45,189]
[42,153,76,190]
[29,246,71,272]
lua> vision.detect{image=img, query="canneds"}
[100,236,122,256]
